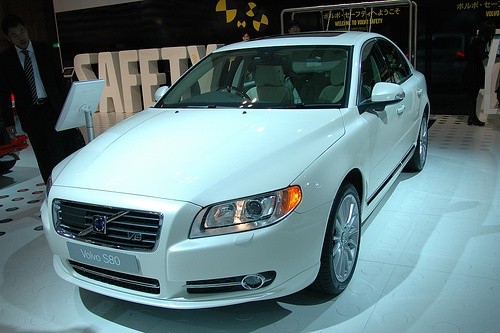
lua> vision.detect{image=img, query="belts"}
[37,98,49,105]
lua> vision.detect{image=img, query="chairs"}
[317,61,371,103]
[242,65,295,104]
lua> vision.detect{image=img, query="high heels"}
[468,115,485,126]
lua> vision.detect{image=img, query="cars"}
[41,31,430,310]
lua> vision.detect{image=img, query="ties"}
[21,50,38,105]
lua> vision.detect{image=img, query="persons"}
[0,14,86,184]
[242,31,250,41]
[288,20,301,32]
[460,19,496,126]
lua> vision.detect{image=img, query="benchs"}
[286,73,330,104]
